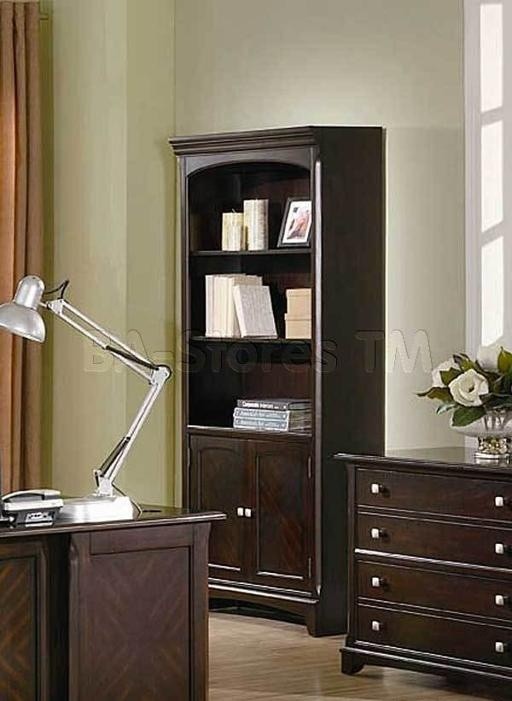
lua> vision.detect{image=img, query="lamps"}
[1,275,172,524]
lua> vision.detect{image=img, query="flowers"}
[415,346,512,435]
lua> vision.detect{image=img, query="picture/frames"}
[276,194,311,249]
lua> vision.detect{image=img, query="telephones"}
[0,489,65,531]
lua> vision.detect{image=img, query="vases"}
[471,433,512,462]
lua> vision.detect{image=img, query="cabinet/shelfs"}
[167,126,384,636]
[330,447,510,701]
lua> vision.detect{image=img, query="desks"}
[0,505,226,701]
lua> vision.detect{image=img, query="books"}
[233,399,312,432]
[205,198,278,340]
